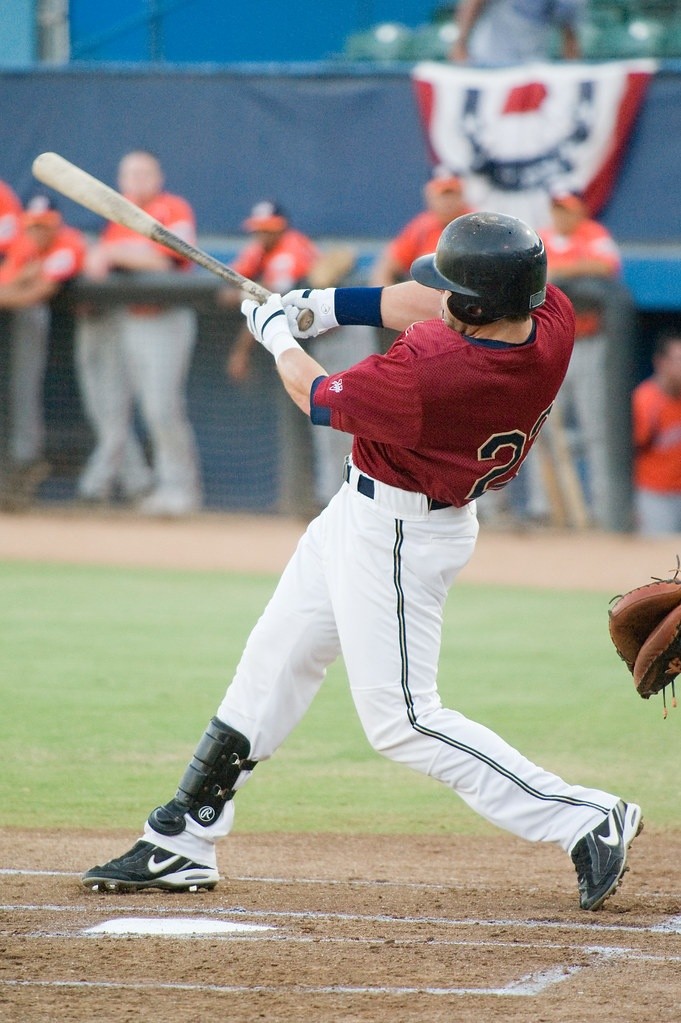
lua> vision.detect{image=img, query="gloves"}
[241,292,293,352]
[282,287,341,339]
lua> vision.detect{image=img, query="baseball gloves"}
[607,580,681,701]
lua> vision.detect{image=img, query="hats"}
[551,189,588,215]
[243,203,288,233]
[424,166,460,196]
[19,193,62,230]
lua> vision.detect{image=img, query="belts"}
[342,455,452,511]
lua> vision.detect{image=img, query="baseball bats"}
[31,150,314,335]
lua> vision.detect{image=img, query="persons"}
[449,0,580,61]
[79,211,644,910]
[0,151,681,534]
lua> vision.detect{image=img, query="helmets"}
[410,212,547,325]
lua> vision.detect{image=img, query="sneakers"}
[571,799,644,910]
[82,840,220,893]
